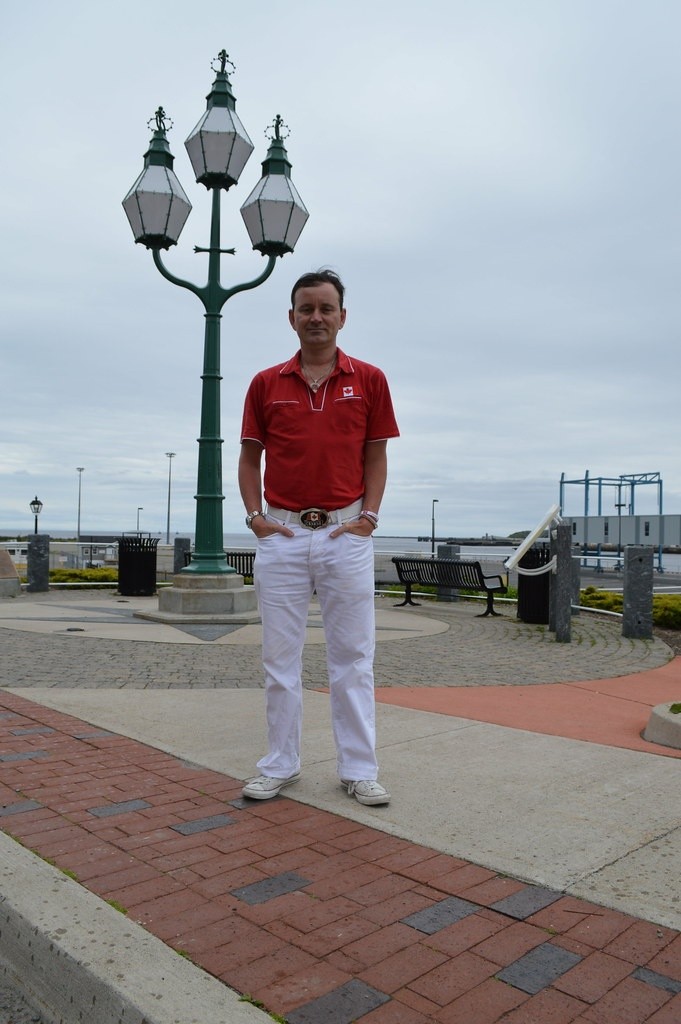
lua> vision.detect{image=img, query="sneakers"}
[340,778,390,805]
[241,775,301,799]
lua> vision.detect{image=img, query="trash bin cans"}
[116,537,161,595]
[513,548,550,624]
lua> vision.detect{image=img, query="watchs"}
[246,510,266,529]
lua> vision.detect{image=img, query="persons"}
[238,270,402,805]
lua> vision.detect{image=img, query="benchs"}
[183,549,256,579]
[391,556,510,618]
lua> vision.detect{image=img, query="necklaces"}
[302,356,335,392]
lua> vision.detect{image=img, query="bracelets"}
[357,509,379,529]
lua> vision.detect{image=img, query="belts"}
[267,504,363,531]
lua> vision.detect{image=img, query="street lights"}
[430,499,439,577]
[164,452,177,544]
[117,46,307,625]
[137,507,144,537]
[28,495,43,534]
[76,466,84,542]
